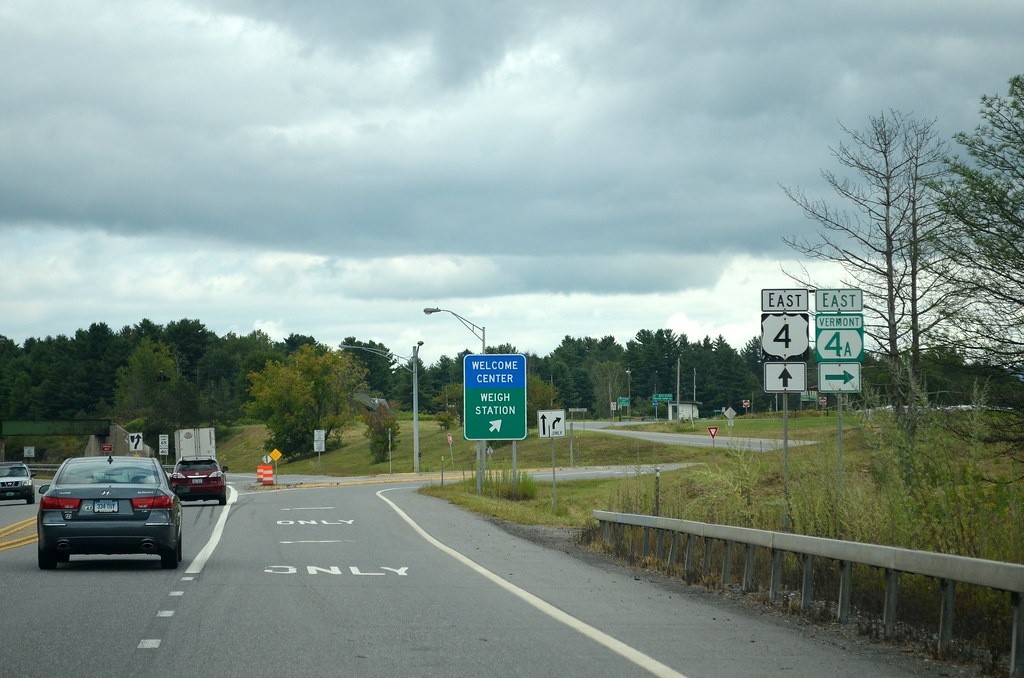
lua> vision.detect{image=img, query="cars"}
[857,405,1014,412]
[36,455,184,570]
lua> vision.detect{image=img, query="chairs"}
[130,475,150,483]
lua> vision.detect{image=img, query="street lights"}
[423,307,487,481]
[339,341,424,473]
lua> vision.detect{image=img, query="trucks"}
[174,428,216,462]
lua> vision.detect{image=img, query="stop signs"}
[819,397,827,405]
[743,399,750,408]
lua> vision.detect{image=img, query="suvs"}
[0,461,36,504]
[168,456,228,505]
